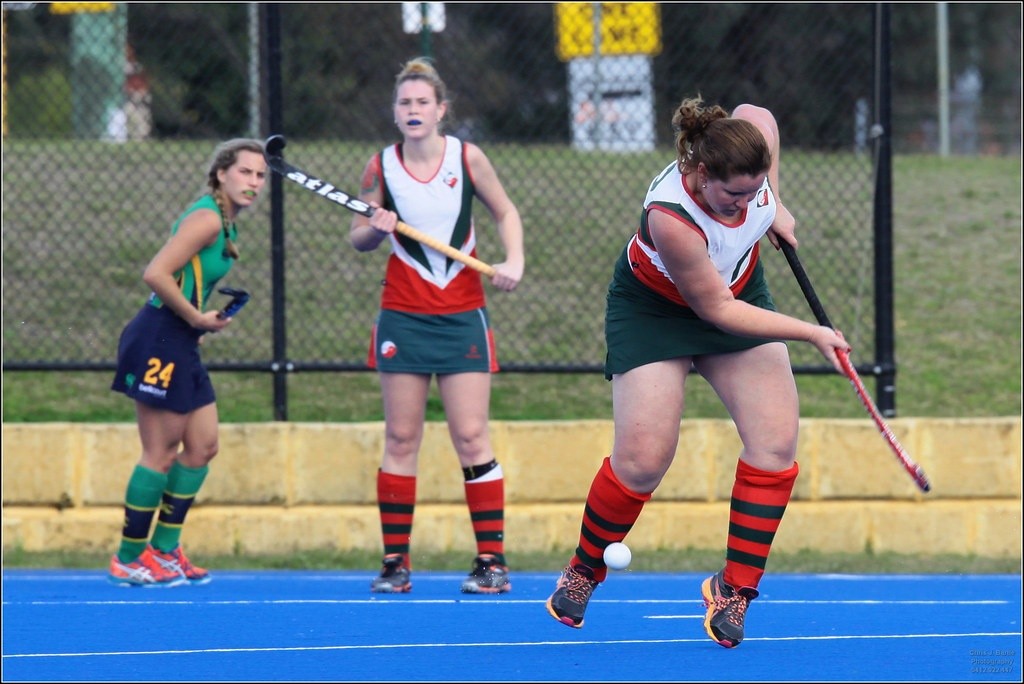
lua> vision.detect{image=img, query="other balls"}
[603,542,632,570]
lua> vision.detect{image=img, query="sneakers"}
[701,569,759,649]
[106,544,212,589]
[370,553,412,593]
[461,556,512,594]
[545,566,599,628]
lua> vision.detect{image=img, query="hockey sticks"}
[213,284,252,320]
[772,231,935,495]
[260,135,495,277]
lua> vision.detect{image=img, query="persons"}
[107,138,267,589]
[350,57,524,592]
[547,98,853,648]
